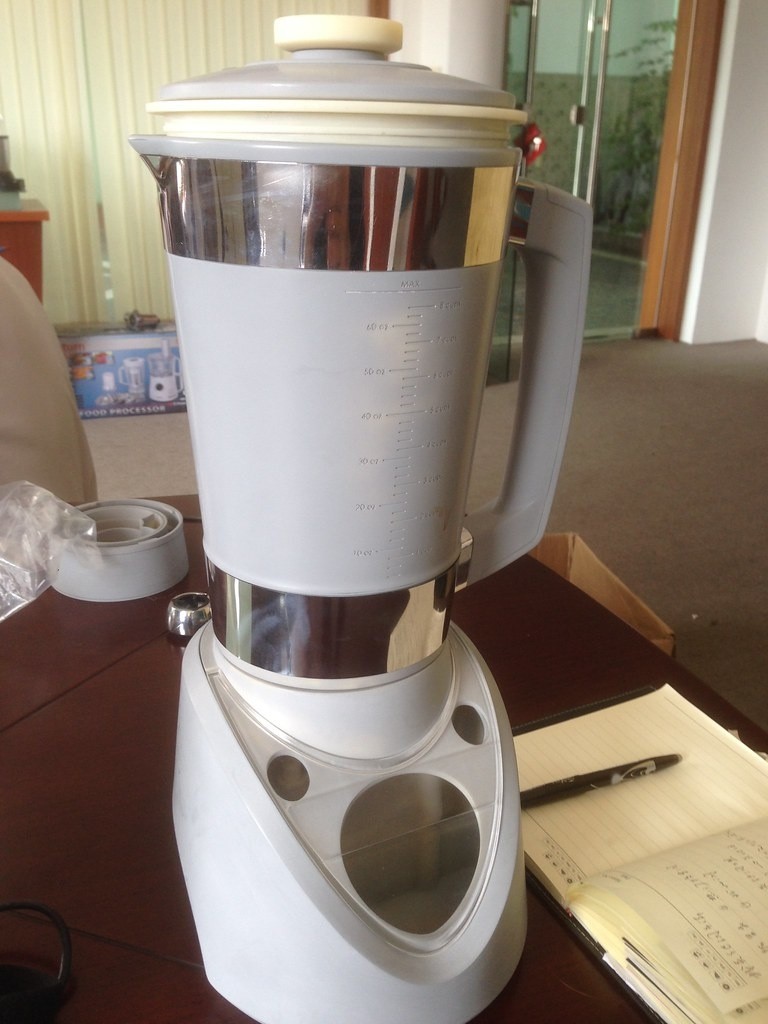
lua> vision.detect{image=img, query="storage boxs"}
[529,531,676,661]
[47,320,187,419]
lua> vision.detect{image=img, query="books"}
[511,682,767,1024]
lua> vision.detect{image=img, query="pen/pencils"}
[520,753,683,811]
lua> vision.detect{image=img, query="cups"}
[102,371,116,390]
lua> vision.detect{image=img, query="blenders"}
[130,15,594,1024]
[119,357,145,402]
[148,351,184,402]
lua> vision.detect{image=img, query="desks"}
[1,495,768,1024]
[0,197,50,304]
